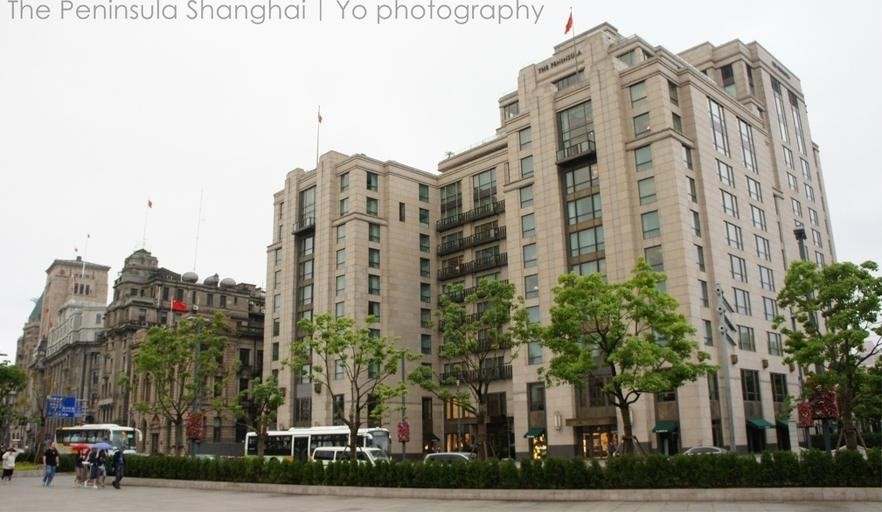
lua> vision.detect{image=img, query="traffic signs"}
[45,395,76,419]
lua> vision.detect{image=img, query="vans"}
[423,452,479,466]
[311,446,391,468]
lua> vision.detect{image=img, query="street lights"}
[792,225,833,457]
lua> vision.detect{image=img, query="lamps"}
[552,409,563,431]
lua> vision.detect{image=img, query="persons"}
[0,440,127,489]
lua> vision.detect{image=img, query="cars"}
[667,446,728,461]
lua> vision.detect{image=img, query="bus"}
[244,425,393,463]
[53,424,143,463]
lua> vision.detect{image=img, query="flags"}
[170,298,187,311]
